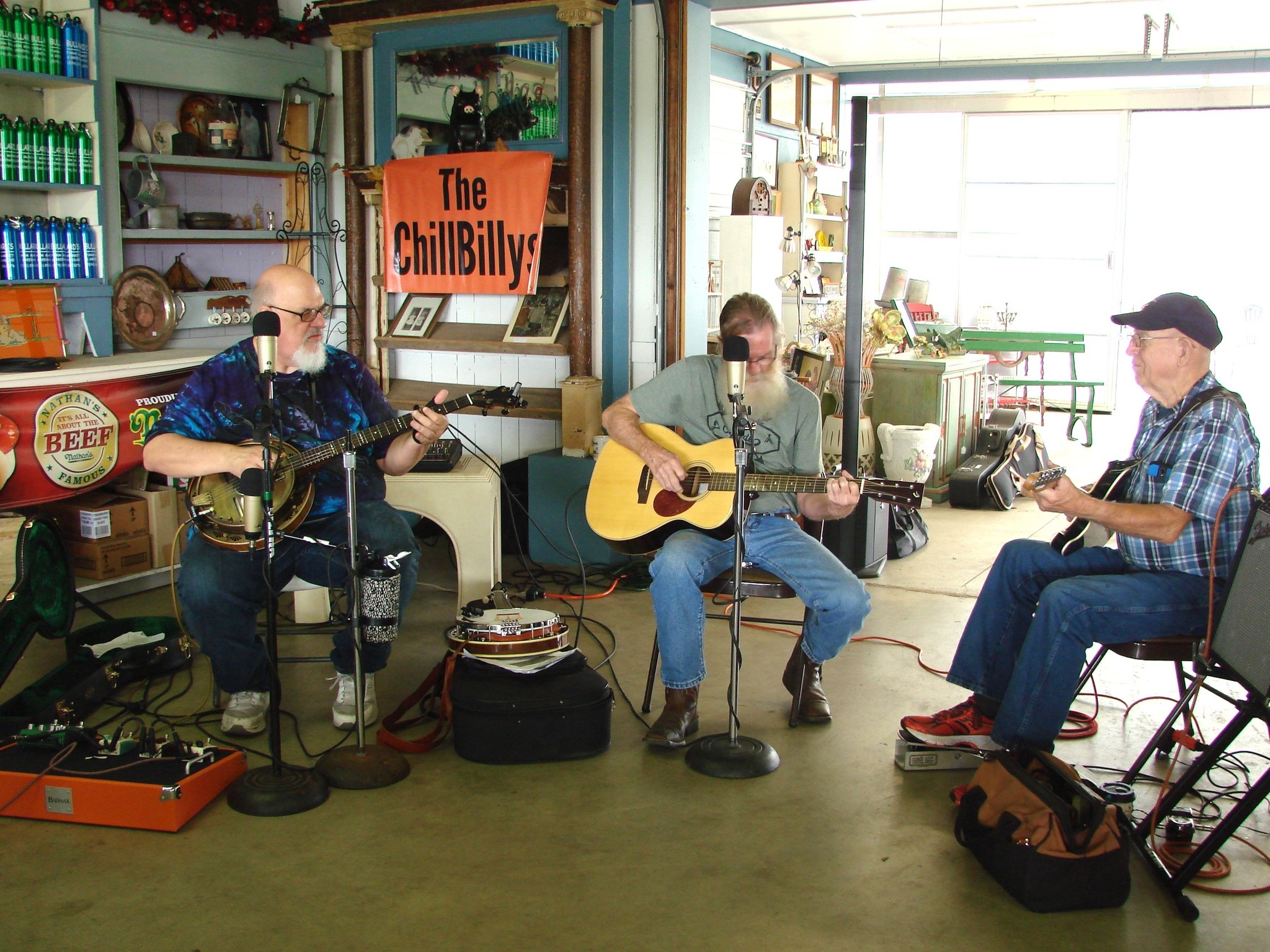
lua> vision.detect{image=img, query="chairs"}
[1070,635,1236,786]
[641,471,826,728]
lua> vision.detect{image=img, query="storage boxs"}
[0,484,193,603]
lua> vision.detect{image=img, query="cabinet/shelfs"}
[778,162,850,351]
[869,351,990,502]
[347,160,570,422]
[396,41,558,147]
[0,0,332,364]
[0,348,223,608]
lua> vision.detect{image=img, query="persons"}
[598,291,872,749]
[143,265,450,736]
[899,292,1261,804]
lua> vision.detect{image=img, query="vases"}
[829,332,878,419]
[876,422,941,509]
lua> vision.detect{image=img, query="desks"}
[296,455,501,624]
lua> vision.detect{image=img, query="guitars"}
[441,587,571,660]
[1022,457,1139,559]
[184,379,529,553]
[581,418,928,557]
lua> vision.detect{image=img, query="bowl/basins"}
[184,211,230,230]
[152,121,179,155]
[147,204,179,230]
[131,119,152,153]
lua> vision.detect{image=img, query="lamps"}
[777,221,822,344]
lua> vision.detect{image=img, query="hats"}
[1109,293,1223,350]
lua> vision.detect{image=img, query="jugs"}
[124,155,166,229]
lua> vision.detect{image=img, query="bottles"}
[497,88,559,140]
[0,2,91,80]
[0,112,94,184]
[0,213,98,280]
[267,211,275,231]
[496,41,556,64]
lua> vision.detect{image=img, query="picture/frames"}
[808,67,838,138]
[503,287,569,344]
[752,131,778,189]
[768,52,802,132]
[228,95,272,163]
[388,293,452,339]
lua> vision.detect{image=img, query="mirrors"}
[372,14,569,167]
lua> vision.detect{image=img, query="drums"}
[454,607,562,642]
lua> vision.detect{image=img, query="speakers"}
[1208,497,1270,707]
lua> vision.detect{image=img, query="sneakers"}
[327,671,378,730]
[949,785,965,805]
[898,692,1001,750]
[220,690,270,735]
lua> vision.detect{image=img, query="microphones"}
[252,311,281,430]
[722,336,749,404]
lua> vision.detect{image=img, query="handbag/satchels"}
[888,505,929,558]
[955,745,1130,910]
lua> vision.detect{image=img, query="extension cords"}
[1164,805,1195,842]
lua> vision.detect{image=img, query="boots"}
[642,685,700,748]
[782,635,833,723]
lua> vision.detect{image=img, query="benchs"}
[959,331,1105,447]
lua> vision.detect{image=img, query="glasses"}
[746,345,777,366]
[1127,334,1195,349]
[269,303,333,323]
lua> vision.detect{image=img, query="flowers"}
[809,301,907,351]
